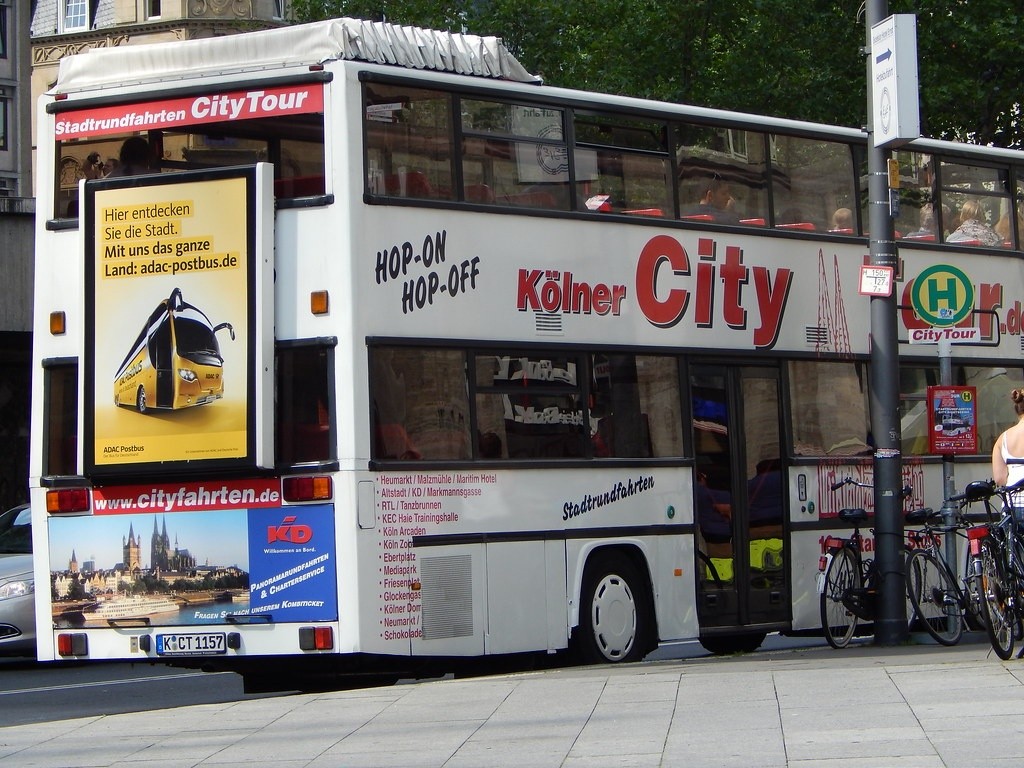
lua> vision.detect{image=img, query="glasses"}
[712,171,721,183]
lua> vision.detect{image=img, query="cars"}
[0,504,37,653]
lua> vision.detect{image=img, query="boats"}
[61,586,181,623]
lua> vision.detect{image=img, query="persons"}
[906,204,949,243]
[689,177,739,225]
[696,459,782,557]
[991,388,1024,548]
[832,207,852,230]
[994,212,1024,249]
[945,200,1005,248]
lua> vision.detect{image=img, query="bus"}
[114,288,235,414]
[29,16,1024,674]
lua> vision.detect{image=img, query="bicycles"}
[816,477,922,649]
[949,478,1024,660]
[905,501,995,646]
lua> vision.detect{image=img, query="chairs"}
[273,170,1012,252]
[297,410,424,469]
